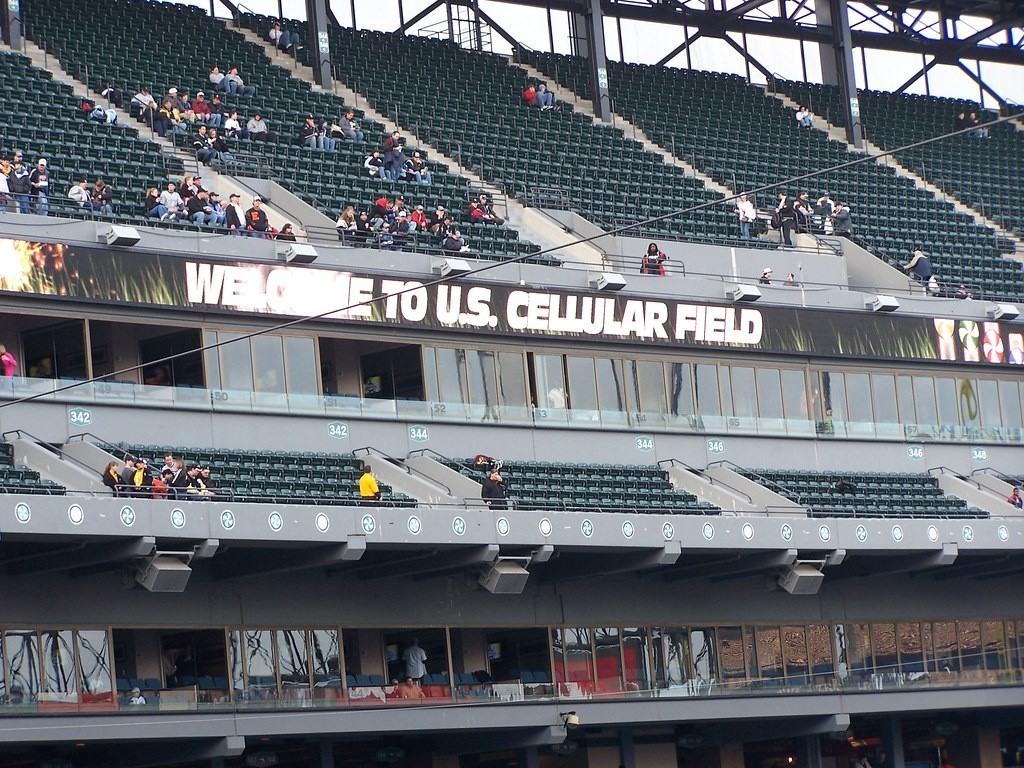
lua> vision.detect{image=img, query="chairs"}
[0,0,1024,302]
[116,670,553,706]
[1,439,1024,519]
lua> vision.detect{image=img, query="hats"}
[306,114,313,119]
[197,91,204,96]
[413,152,420,159]
[132,687,140,693]
[392,679,399,685]
[123,453,133,461]
[762,268,773,274]
[822,190,829,195]
[16,150,23,157]
[359,188,497,238]
[364,465,371,473]
[168,87,177,94]
[186,460,200,468]
[142,86,150,92]
[834,201,847,207]
[201,462,210,469]
[134,457,143,467]
[190,173,263,206]
[39,158,46,166]
[79,174,88,183]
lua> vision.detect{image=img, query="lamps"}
[993,304,1020,321]
[559,710,581,729]
[597,273,626,292]
[103,226,142,247]
[733,285,762,302]
[872,296,900,313]
[440,258,472,278]
[284,243,319,263]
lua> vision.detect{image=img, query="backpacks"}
[771,212,783,229]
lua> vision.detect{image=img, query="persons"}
[523,380,568,421]
[130,64,269,166]
[800,387,833,421]
[904,249,932,287]
[942,659,954,671]
[145,175,297,242]
[102,453,216,501]
[758,267,800,287]
[522,84,554,111]
[269,22,304,50]
[640,243,666,276]
[359,466,381,507]
[735,191,855,248]
[957,111,989,139]
[144,367,171,385]
[0,344,17,378]
[932,285,974,300]
[387,636,428,701]
[795,105,813,128]
[297,109,432,185]
[854,751,889,768]
[130,687,145,704]
[0,151,113,216]
[481,469,509,510]
[336,193,503,255]
[1008,488,1023,509]
